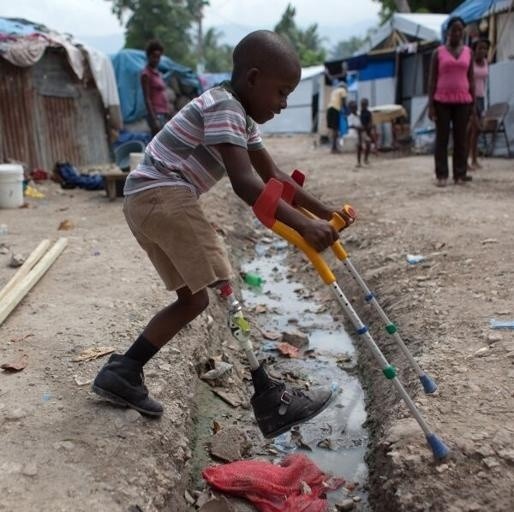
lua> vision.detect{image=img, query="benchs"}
[100,173,129,201]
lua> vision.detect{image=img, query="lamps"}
[479,18,489,33]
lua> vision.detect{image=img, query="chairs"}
[477,102,513,158]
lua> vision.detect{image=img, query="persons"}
[465,39,489,170]
[360,97,378,157]
[326,81,349,153]
[348,101,370,167]
[93,30,350,439]
[140,39,170,137]
[427,17,476,186]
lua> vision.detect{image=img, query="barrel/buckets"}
[129,153,145,170]
[0,163,24,208]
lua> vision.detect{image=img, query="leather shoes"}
[251,383,334,439]
[90,353,163,418]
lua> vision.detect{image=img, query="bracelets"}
[428,104,434,107]
